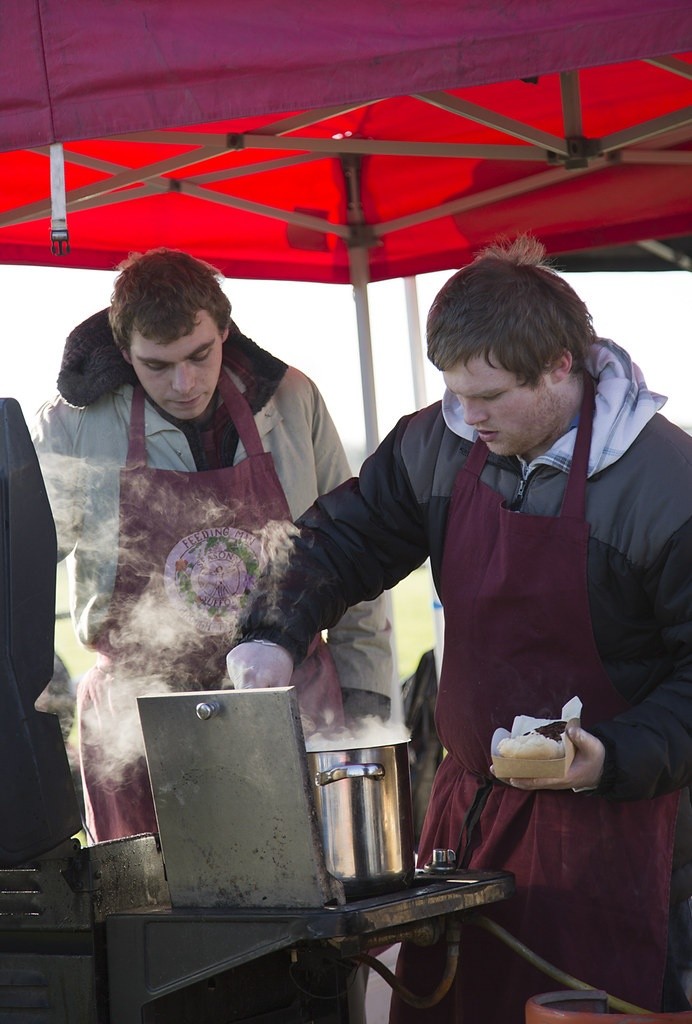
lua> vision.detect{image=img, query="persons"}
[225,231,692,1024]
[28,244,394,835]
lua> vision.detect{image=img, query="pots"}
[306,735,415,898]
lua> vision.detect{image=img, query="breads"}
[497,732,560,760]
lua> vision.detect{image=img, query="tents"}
[0,0,692,669]
